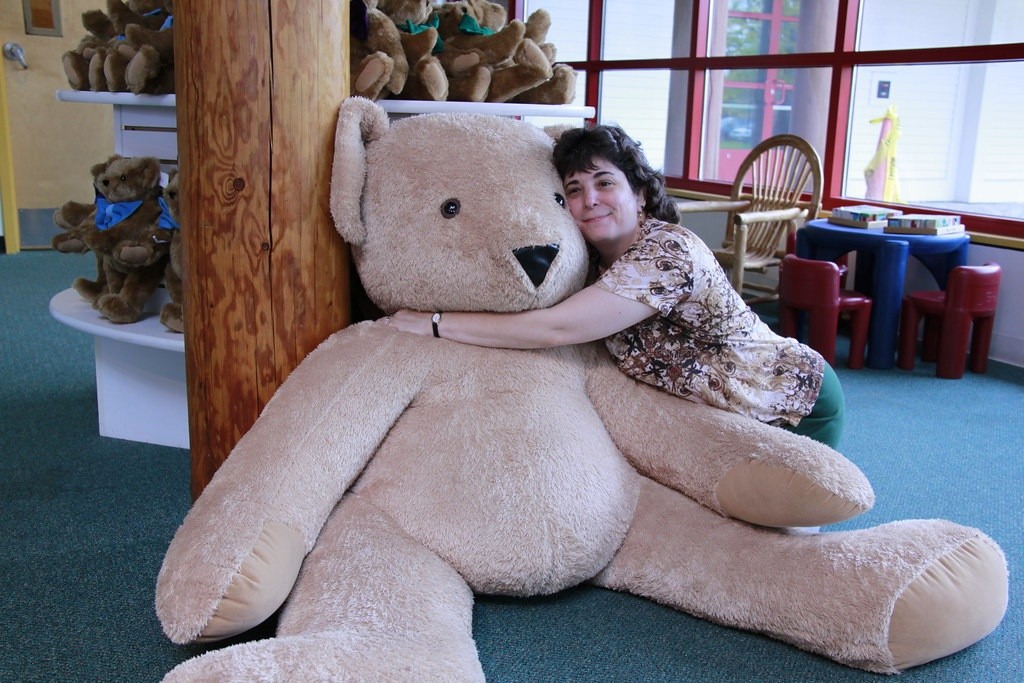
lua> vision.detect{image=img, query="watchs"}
[432,312,443,338]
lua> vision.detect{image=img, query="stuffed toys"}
[351,0,577,104]
[153,96,1008,683]
[52,154,186,334]
[62,1,175,96]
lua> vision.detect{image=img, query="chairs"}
[666,135,824,305]
[897,262,1001,379]
[780,255,872,369]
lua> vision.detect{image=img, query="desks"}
[807,217,971,371]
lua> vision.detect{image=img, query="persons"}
[386,125,844,533]
